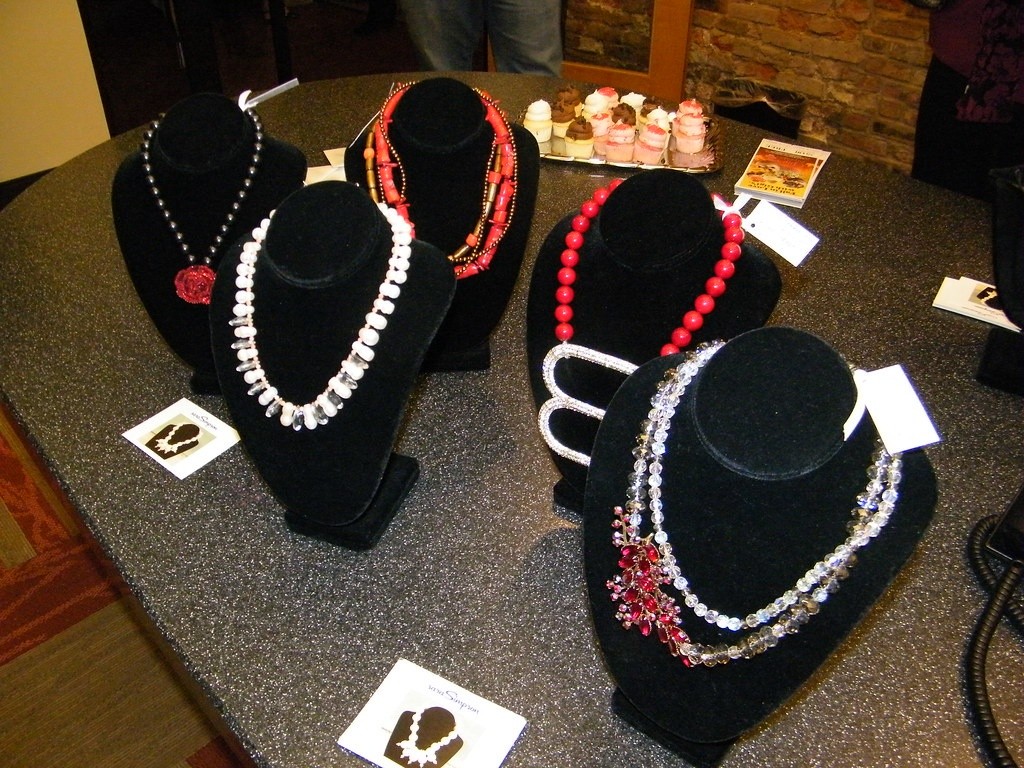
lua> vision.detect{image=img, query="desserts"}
[522,87,706,164]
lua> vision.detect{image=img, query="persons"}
[403,0,564,77]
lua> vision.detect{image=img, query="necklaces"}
[607,340,903,668]
[363,78,517,278]
[140,107,261,304]
[537,176,744,468]
[230,203,411,431]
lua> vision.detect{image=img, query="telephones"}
[981,480,1024,568]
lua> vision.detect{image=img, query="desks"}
[0,68,1024,768]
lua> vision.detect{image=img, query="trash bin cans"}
[712,75,809,141]
